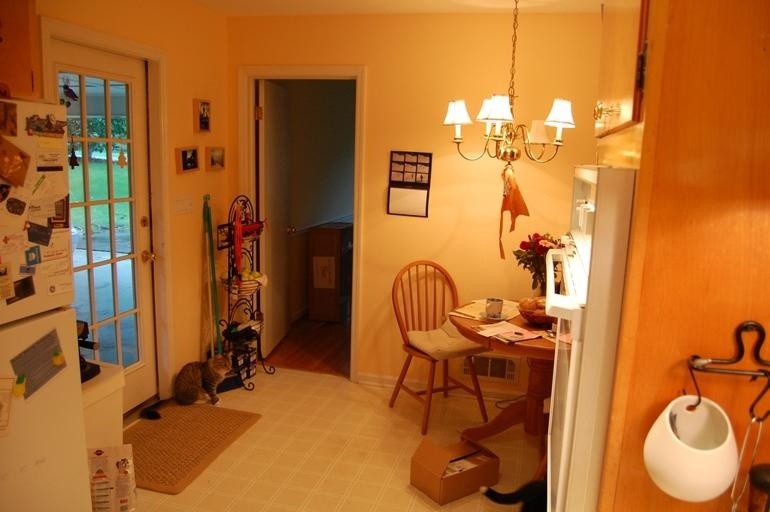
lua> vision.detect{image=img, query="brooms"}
[203,201,242,395]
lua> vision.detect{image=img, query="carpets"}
[123,400,264,495]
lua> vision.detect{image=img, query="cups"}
[486,298,503,318]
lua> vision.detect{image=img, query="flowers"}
[512,233,565,297]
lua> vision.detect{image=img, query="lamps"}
[443,0,576,259]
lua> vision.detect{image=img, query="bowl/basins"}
[517,306,559,327]
[219,275,267,298]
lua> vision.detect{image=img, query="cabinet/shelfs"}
[80,357,125,449]
[308,222,352,323]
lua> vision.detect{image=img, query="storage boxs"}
[410,435,499,506]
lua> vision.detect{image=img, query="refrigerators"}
[0,95,94,512]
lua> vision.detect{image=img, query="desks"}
[449,297,572,441]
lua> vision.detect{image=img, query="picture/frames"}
[204,144,225,172]
[192,97,213,135]
[175,146,201,175]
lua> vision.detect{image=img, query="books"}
[472,320,544,345]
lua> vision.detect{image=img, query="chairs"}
[391,260,488,436]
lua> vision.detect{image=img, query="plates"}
[478,313,508,321]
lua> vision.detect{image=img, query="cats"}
[140,348,236,420]
[479,478,547,512]
